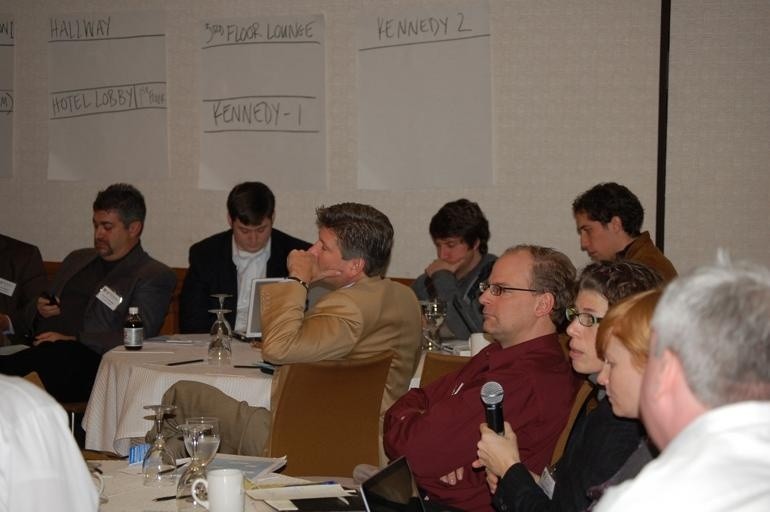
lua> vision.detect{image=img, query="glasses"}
[566,305,602,327]
[479,283,538,296]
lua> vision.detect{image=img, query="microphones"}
[480,382,505,438]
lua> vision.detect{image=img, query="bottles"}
[123,306,144,350]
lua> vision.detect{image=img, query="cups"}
[467,333,493,359]
[190,468,244,511]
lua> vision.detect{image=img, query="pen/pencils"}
[163,340,192,344]
[93,467,103,474]
[234,365,262,368]
[286,480,337,486]
[167,359,204,366]
[153,492,208,502]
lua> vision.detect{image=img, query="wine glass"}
[141,404,220,512]
[422,300,447,351]
[208,292,233,368]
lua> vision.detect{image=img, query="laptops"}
[232,277,284,342]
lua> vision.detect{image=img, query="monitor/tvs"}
[360,455,425,512]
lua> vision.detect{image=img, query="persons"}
[176,181,315,335]
[1,375,101,512]
[132,202,422,479]
[1,184,175,402]
[382,182,770,512]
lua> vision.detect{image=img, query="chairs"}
[419,351,473,392]
[549,376,593,471]
[268,351,395,482]
[60,402,88,441]
